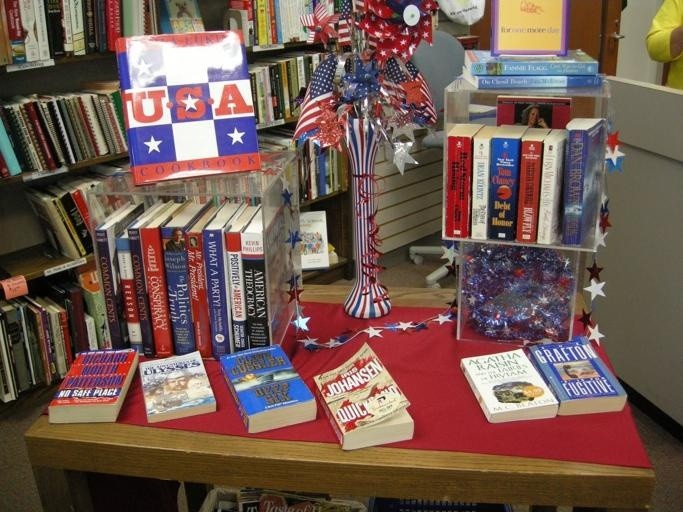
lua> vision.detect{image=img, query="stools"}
[410,131,454,290]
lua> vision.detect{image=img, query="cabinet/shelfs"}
[442,78,610,344]
[0,0,350,409]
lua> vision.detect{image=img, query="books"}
[0,199,339,402]
[0,51,349,260]
[524,336,626,416]
[139,350,216,423]
[313,343,415,450]
[1,1,344,71]
[48,346,139,423]
[460,348,559,423]
[221,344,317,433]
[446,35,607,245]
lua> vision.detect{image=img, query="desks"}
[25,285,656,512]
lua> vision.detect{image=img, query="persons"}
[645,0,683,90]
